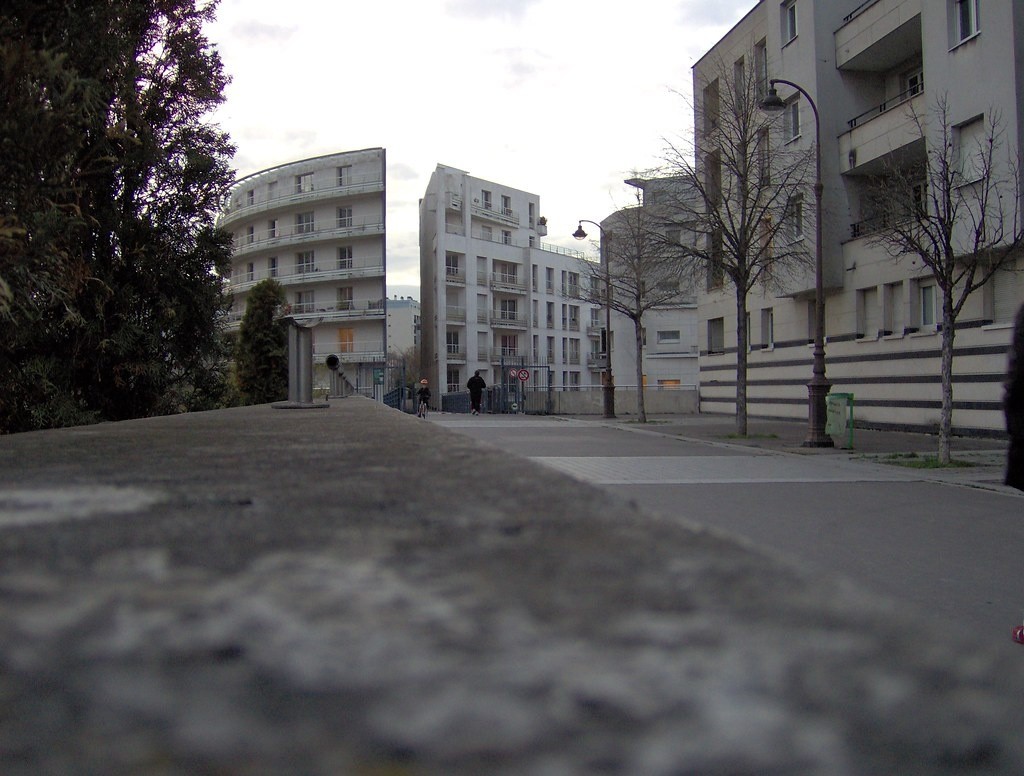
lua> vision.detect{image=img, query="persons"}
[415,379,431,418]
[467,370,486,415]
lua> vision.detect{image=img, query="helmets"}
[421,379,428,383]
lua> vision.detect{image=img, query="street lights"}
[573,218,617,418]
[759,79,836,449]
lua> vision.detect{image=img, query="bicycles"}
[414,393,428,419]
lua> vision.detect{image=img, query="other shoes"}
[472,410,476,415]
[476,414,478,415]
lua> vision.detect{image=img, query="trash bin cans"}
[825,392,851,435]
